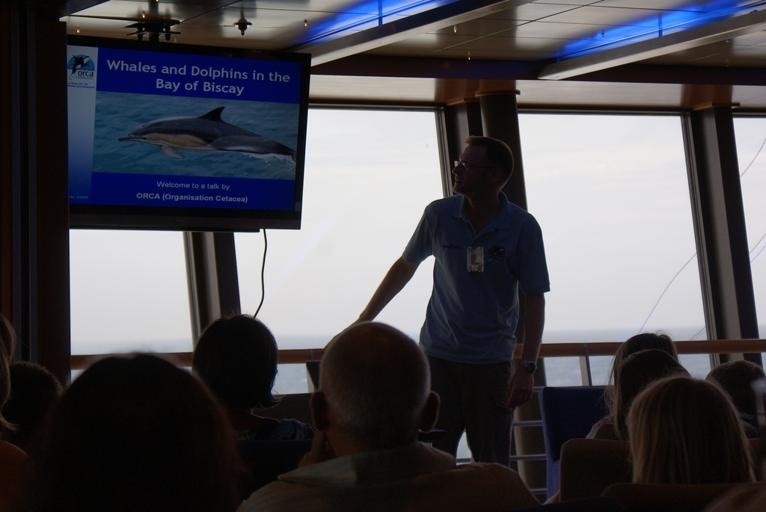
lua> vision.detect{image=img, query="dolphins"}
[116,106,296,164]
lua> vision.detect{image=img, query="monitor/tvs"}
[65,33,312,230]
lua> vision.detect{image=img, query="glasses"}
[451,160,499,170]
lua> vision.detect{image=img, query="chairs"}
[283,438,766,512]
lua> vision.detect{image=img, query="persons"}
[0,315,241,512]
[352,135,552,467]
[585,333,765,512]
[233,323,538,512]
[193,314,313,499]
